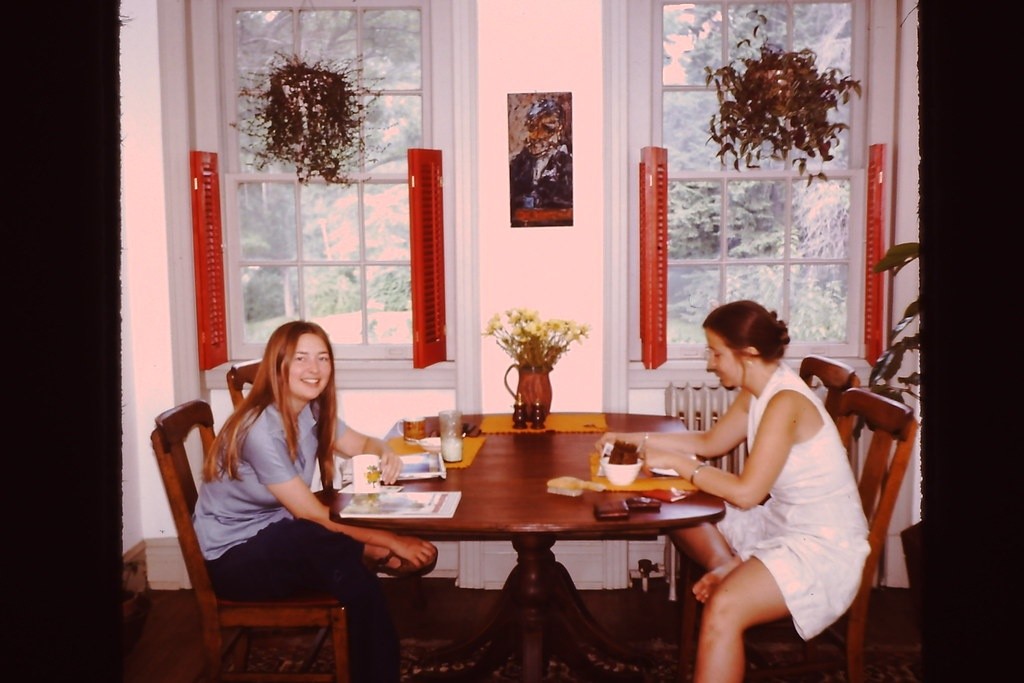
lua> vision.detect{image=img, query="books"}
[597,442,697,477]
[337,443,462,518]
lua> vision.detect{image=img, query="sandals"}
[365,545,438,577]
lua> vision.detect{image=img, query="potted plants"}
[230,49,390,190]
[704,9,863,186]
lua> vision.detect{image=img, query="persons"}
[192,321,439,683]
[595,300,871,683]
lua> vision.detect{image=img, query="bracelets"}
[690,463,709,484]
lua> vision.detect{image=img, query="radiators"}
[665,382,888,602]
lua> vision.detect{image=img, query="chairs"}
[150,365,350,683]
[676,357,919,683]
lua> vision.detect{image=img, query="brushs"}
[547,476,604,498]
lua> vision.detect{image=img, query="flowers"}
[480,307,587,368]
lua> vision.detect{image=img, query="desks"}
[327,412,725,683]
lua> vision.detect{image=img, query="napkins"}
[386,436,488,469]
[480,414,607,433]
[589,453,700,491]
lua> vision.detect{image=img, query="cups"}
[351,455,383,494]
[397,416,427,445]
[439,408,463,465]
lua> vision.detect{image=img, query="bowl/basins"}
[420,437,444,453]
[599,458,644,488]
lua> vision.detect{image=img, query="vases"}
[504,364,553,421]
